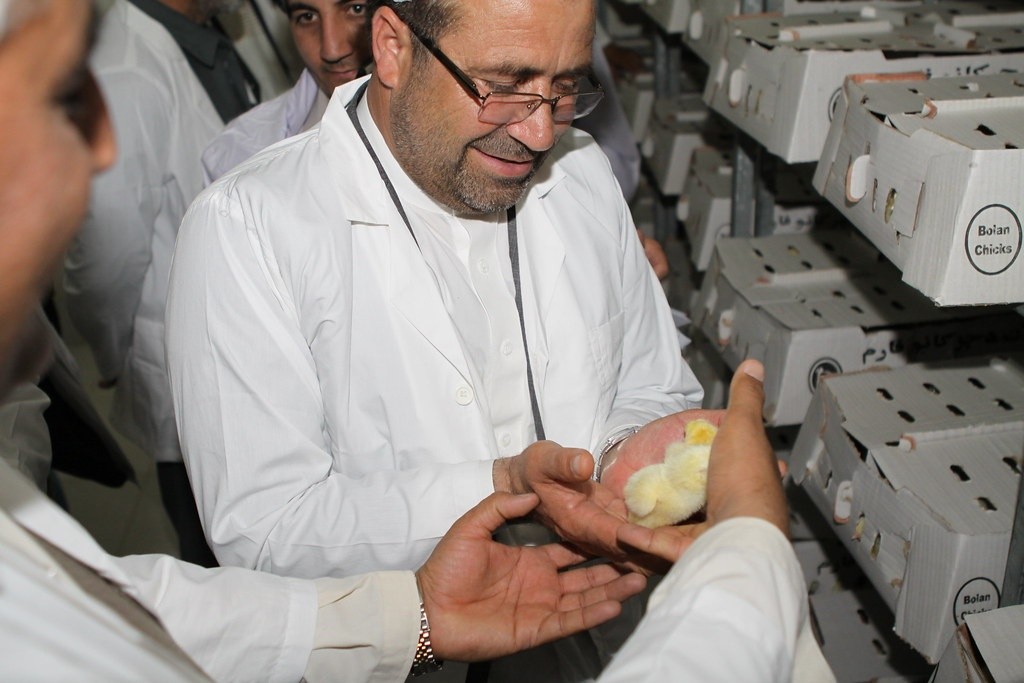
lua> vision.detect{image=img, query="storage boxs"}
[588,0,1023,683]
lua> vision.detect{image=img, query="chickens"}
[619,416,721,531]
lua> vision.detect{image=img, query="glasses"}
[386,3,606,125]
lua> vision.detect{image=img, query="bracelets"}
[410,593,443,677]
[592,427,641,482]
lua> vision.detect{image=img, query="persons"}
[165,0,787,683]
[108,0,675,572]
[52,0,309,567]
[0,0,839,683]
